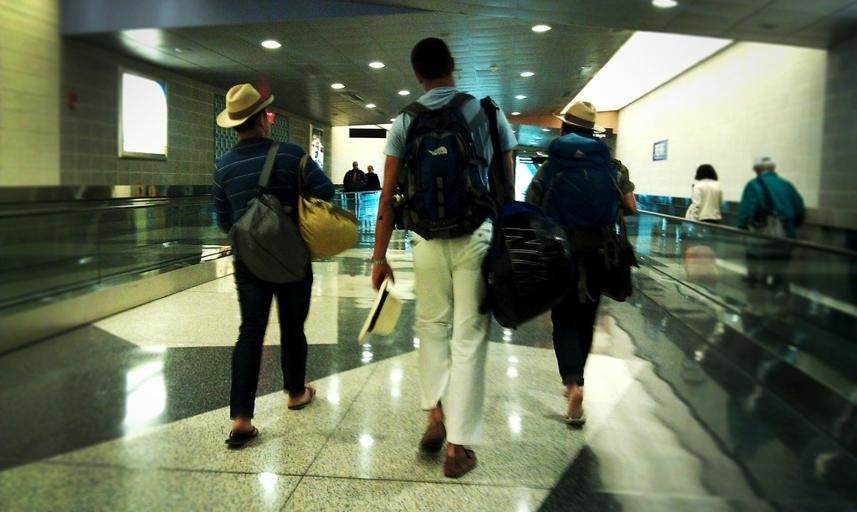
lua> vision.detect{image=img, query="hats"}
[549,99,598,132]
[215,82,274,129]
[358,273,403,345]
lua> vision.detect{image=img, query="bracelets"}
[370,258,387,265]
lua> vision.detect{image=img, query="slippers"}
[287,384,314,410]
[225,424,257,447]
[562,415,586,426]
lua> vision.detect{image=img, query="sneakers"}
[421,420,447,452]
[444,441,476,478]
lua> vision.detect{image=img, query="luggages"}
[683,243,720,285]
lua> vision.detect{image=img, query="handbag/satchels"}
[297,192,362,257]
[228,193,310,283]
[585,230,637,304]
[748,212,789,261]
[488,197,568,330]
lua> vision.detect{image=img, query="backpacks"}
[398,92,489,240]
[551,130,618,225]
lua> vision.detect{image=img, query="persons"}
[373,38,518,478]
[344,161,367,210]
[365,165,380,191]
[738,156,808,319]
[685,164,723,251]
[523,101,636,424]
[213,84,335,445]
